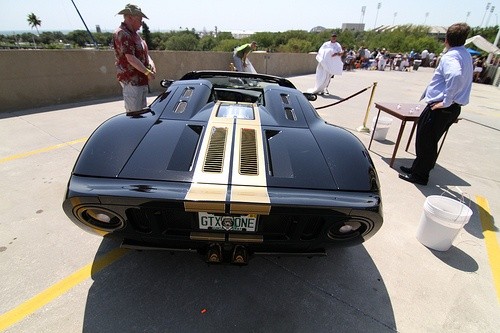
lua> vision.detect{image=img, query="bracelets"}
[145,70,151,75]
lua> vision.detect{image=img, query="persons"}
[421,48,437,66]
[113,4,156,112]
[399,48,417,71]
[342,45,390,70]
[316,33,345,95]
[233,41,257,72]
[399,23,473,186]
[473,55,485,81]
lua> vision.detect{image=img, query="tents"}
[464,35,500,83]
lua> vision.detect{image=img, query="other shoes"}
[400,165,412,174]
[321,93,324,95]
[399,173,422,185]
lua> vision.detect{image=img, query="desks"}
[368,101,450,168]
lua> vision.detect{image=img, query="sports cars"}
[62,70,384,267]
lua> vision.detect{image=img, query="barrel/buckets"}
[416,186,474,252]
[371,116,392,141]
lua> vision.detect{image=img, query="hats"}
[332,31,337,36]
[118,4,148,19]
